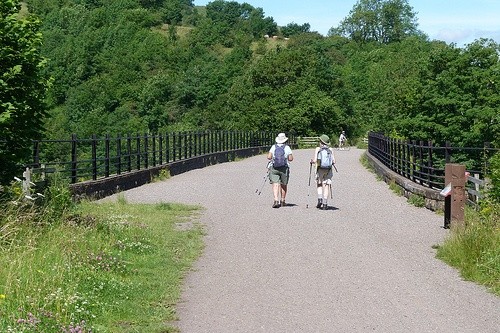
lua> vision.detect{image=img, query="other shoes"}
[280,201,286,207]
[316,199,323,208]
[272,201,280,208]
[324,204,328,210]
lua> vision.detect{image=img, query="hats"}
[275,133,288,143]
[318,134,331,145]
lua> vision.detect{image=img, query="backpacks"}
[317,147,332,169]
[273,143,286,168]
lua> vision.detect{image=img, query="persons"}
[312,135,336,209]
[267,133,293,207]
[340,131,346,147]
